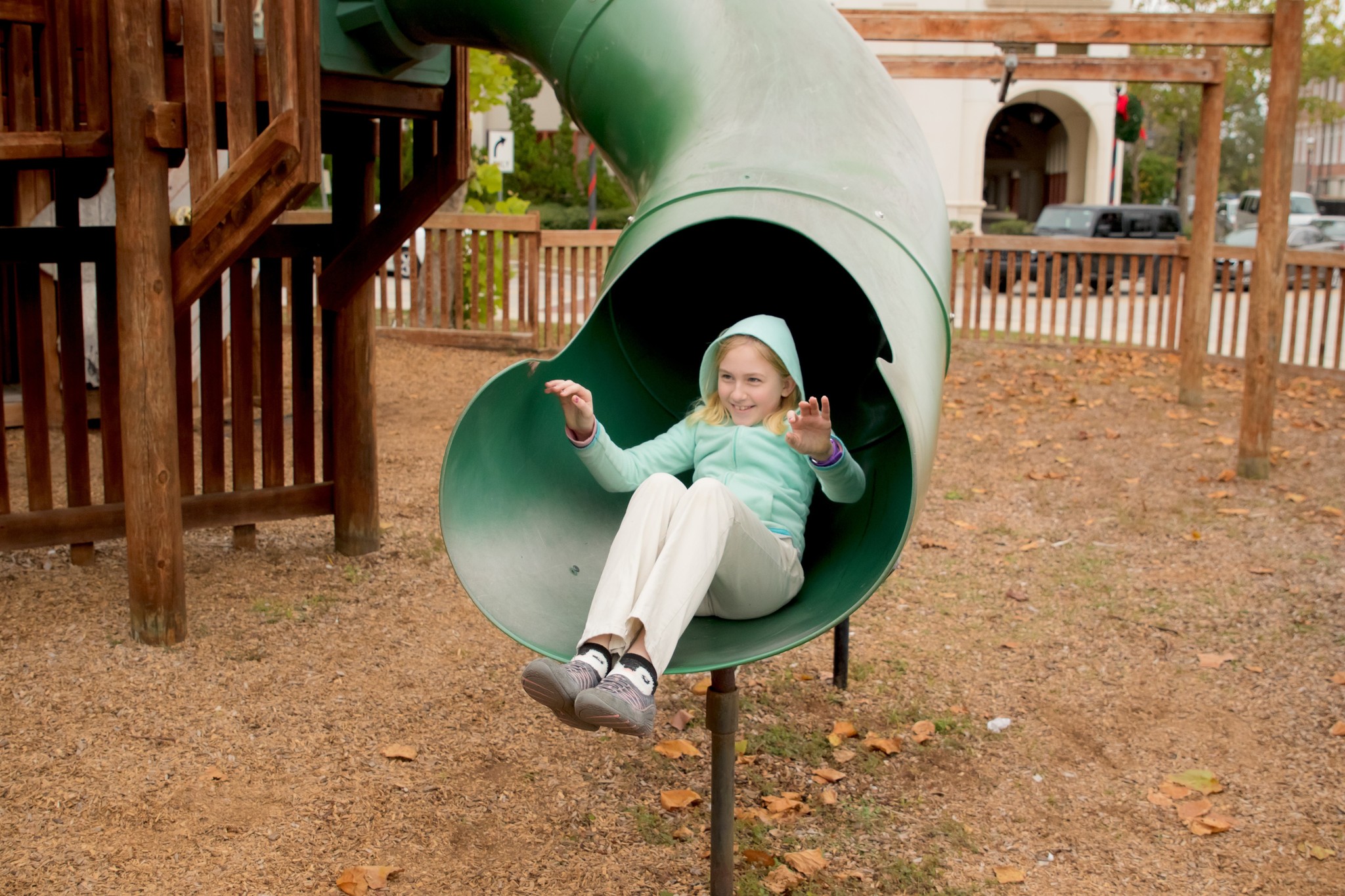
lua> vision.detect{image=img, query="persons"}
[521,315,866,740]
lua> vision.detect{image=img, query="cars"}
[371,203,490,278]
[1213,222,1343,292]
[1307,215,1345,263]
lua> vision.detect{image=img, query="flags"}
[1113,91,1146,144]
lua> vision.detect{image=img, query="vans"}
[1237,191,1320,230]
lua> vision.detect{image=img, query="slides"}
[331,0,954,674]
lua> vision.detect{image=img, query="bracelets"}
[810,437,843,468]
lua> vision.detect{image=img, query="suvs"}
[981,203,1187,298]
[1217,199,1243,233]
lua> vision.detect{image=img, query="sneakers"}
[573,673,657,738]
[520,657,604,732]
